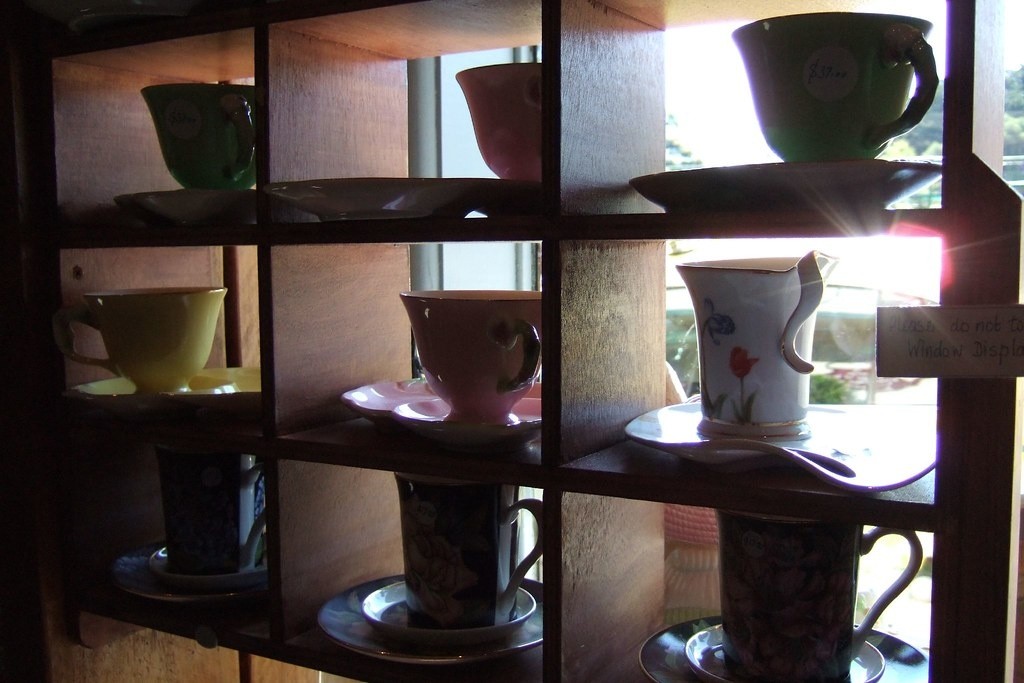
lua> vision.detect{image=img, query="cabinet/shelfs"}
[0,0,1024,683]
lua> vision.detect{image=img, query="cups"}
[674,253,842,442]
[139,83,259,188]
[401,289,542,418]
[153,443,266,575]
[457,63,541,178]
[710,508,922,683]
[391,468,543,627]
[53,283,229,392]
[740,12,938,162]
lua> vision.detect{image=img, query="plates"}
[71,366,262,423]
[628,163,941,216]
[360,578,537,647]
[624,402,938,493]
[636,615,929,683]
[108,537,270,607]
[113,189,256,225]
[339,380,543,449]
[19,0,207,32]
[685,623,886,683]
[317,572,544,668]
[145,545,268,591]
[262,176,542,220]
